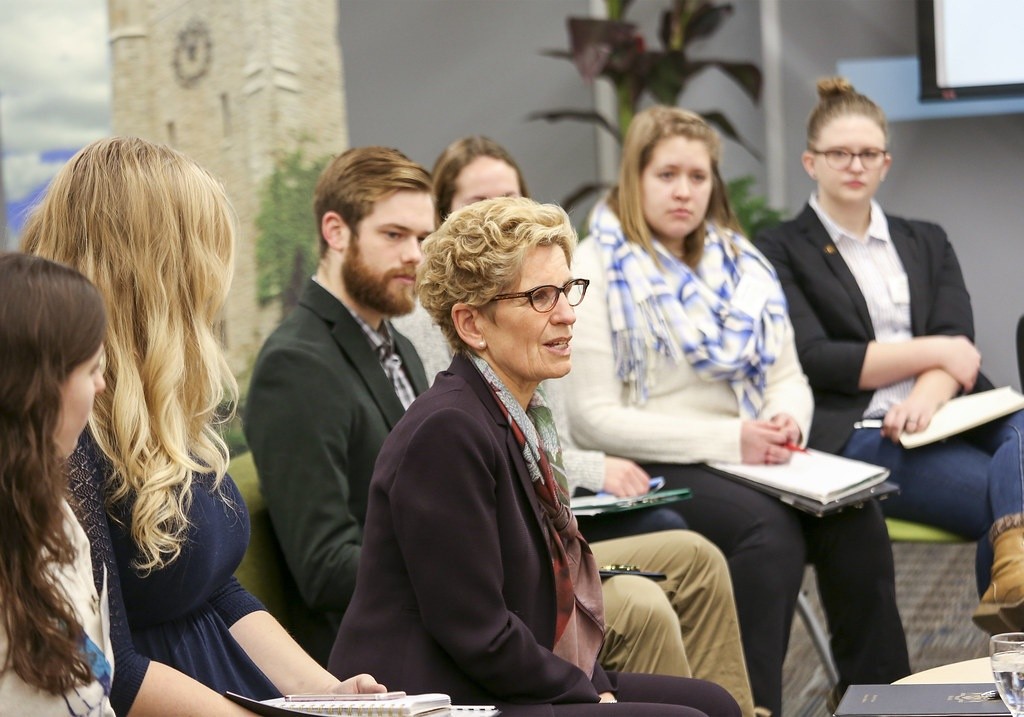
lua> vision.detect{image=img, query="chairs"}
[224,447,322,666]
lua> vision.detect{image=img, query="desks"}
[892,648,1021,684]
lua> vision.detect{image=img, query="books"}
[599,569,667,582]
[833,683,1012,717]
[702,446,900,517]
[899,386,1024,449]
[224,691,500,717]
[570,476,691,516]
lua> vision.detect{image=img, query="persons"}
[761,77,1024,639]
[557,107,912,717]
[0,252,116,717]
[391,134,688,545]
[19,138,386,717]
[327,197,743,717]
[245,145,755,717]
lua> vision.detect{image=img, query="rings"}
[765,448,771,464]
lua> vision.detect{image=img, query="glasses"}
[812,146,889,170]
[488,278,590,313]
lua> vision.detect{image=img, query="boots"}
[971,530,1024,633]
